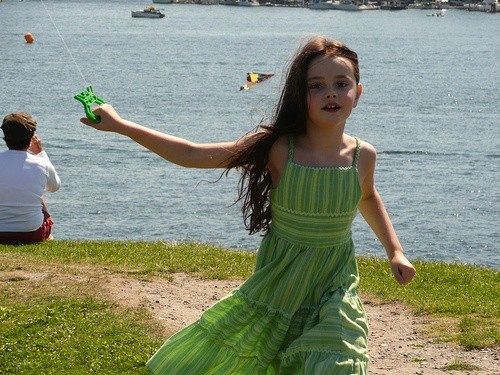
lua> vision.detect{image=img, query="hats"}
[0,112,37,140]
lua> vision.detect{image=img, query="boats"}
[236,0,408,11]
[131,5,167,19]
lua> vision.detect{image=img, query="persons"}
[0,111,61,242]
[80,34,416,375]
